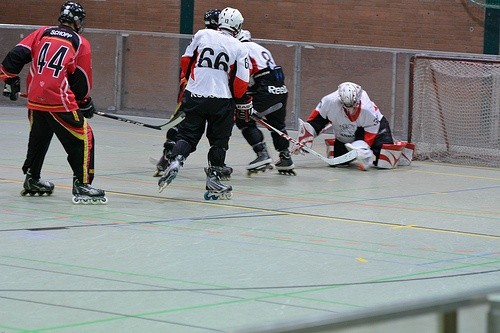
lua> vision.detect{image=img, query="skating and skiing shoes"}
[20,173,53,196]
[72,175,108,204]
[245,147,273,174]
[275,150,297,176]
[157,154,185,193]
[203,166,232,200]
[153,140,176,174]
[219,163,232,180]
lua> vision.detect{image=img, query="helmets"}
[235,29,252,42]
[338,81,362,108]
[59,1,86,34]
[204,8,222,30]
[218,7,244,38]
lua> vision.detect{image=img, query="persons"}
[232,28,296,173]
[290,81,400,170]
[157,5,251,195]
[157,7,236,178]
[1,1,110,198]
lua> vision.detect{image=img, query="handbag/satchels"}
[271,65,285,84]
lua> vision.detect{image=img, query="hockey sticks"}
[249,103,358,167]
[5,85,186,132]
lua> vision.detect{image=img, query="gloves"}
[78,98,95,119]
[345,139,375,172]
[236,95,253,123]
[4,76,21,100]
[291,118,314,156]
